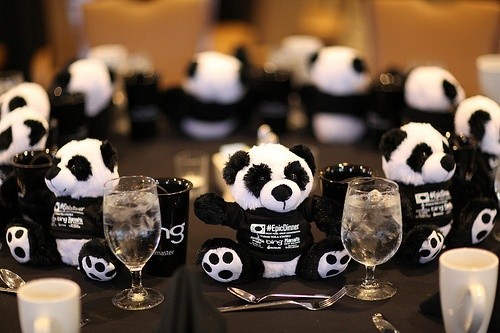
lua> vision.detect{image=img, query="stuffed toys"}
[5,138,120,281]
[54,58,115,138]
[396,65,464,136]
[0,83,50,221]
[193,143,352,287]
[380,122,500,272]
[299,46,376,145]
[454,94,500,196]
[161,51,251,141]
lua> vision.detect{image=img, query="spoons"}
[226,287,332,304]
[0,268,26,289]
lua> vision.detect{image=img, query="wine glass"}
[340,176,403,301]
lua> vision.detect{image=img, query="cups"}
[10,150,61,212]
[318,162,375,223]
[17,277,81,333]
[438,247,499,333]
[134,177,193,278]
[102,176,165,311]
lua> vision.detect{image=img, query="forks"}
[215,286,347,313]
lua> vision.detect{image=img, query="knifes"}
[371,312,400,333]
